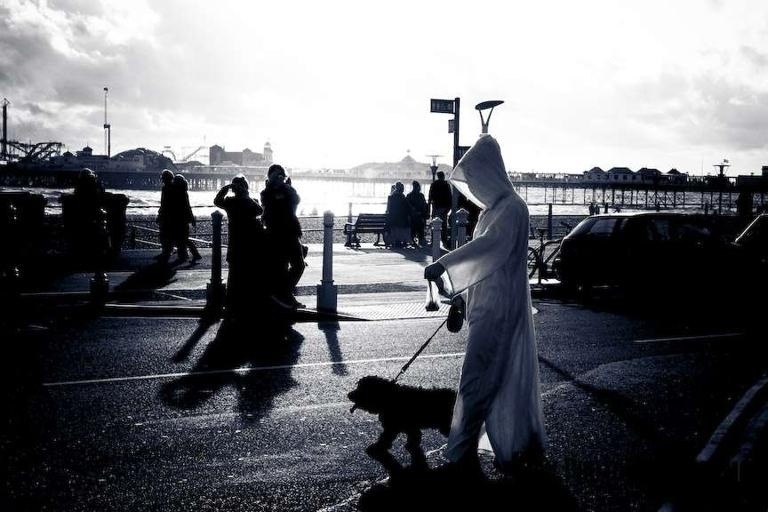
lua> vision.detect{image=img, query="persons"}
[588,203,595,215]
[427,171,451,249]
[72,168,105,269]
[212,174,273,319]
[422,133,550,472]
[97,189,130,265]
[172,174,203,265]
[382,179,429,251]
[594,203,600,214]
[604,203,609,213]
[259,164,306,311]
[152,171,176,262]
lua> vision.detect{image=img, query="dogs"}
[347,376,458,452]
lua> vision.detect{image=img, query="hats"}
[268,165,287,177]
[232,177,248,192]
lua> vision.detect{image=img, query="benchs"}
[343,213,388,248]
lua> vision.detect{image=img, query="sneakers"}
[189,254,202,263]
[422,454,481,474]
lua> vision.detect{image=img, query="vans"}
[552,212,750,311]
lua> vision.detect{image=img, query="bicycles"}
[526,221,574,281]
